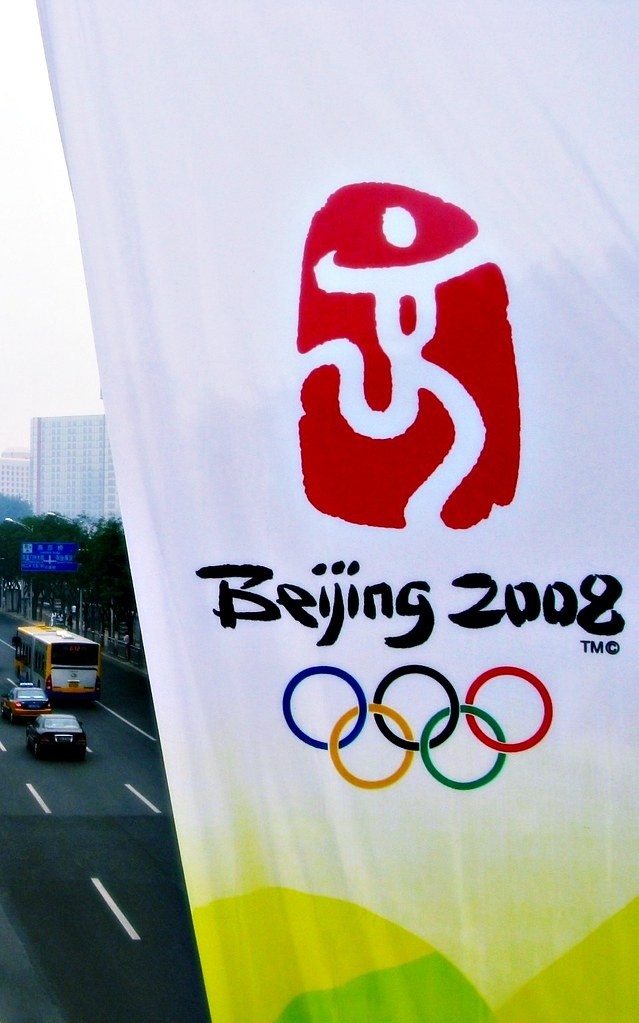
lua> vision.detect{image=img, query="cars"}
[0,683,52,721]
[24,714,86,758]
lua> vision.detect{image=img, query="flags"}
[36,0,639,1023]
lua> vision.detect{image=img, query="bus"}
[11,623,102,702]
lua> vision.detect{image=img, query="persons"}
[51,611,73,632]
[71,604,76,620]
[124,632,130,657]
